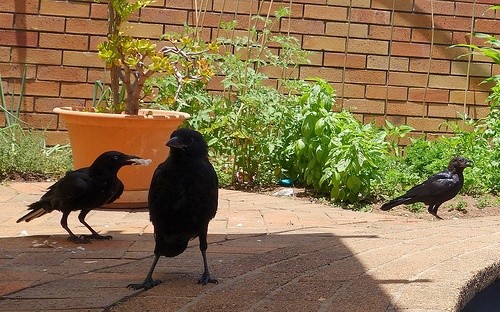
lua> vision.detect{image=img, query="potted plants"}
[53,0,190,212]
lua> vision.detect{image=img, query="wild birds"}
[16,151,150,245]
[125,129,218,290]
[379,157,474,221]
